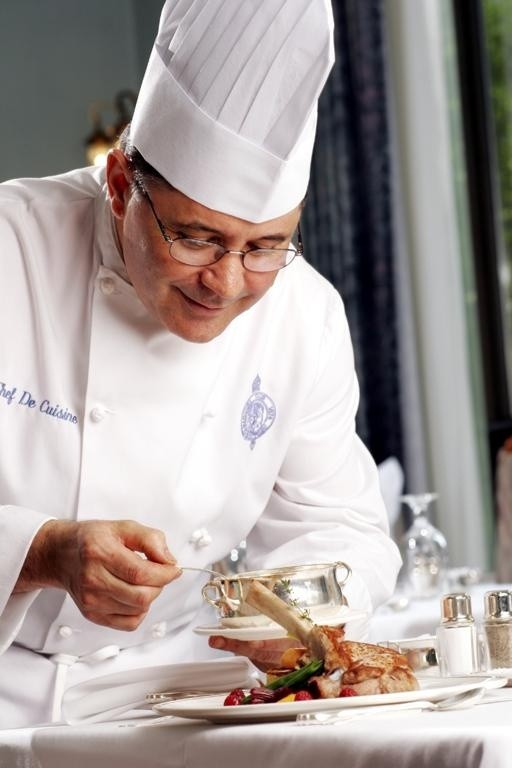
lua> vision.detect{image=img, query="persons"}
[0,1,405,745]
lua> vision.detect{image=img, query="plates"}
[153,678,507,725]
[193,606,373,643]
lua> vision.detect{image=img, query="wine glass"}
[396,491,452,599]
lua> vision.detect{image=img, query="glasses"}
[130,159,304,271]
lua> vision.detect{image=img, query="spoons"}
[298,686,491,727]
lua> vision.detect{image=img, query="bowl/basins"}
[203,559,352,626]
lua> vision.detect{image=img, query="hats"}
[131,0,335,223]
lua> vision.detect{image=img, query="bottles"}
[436,586,511,675]
